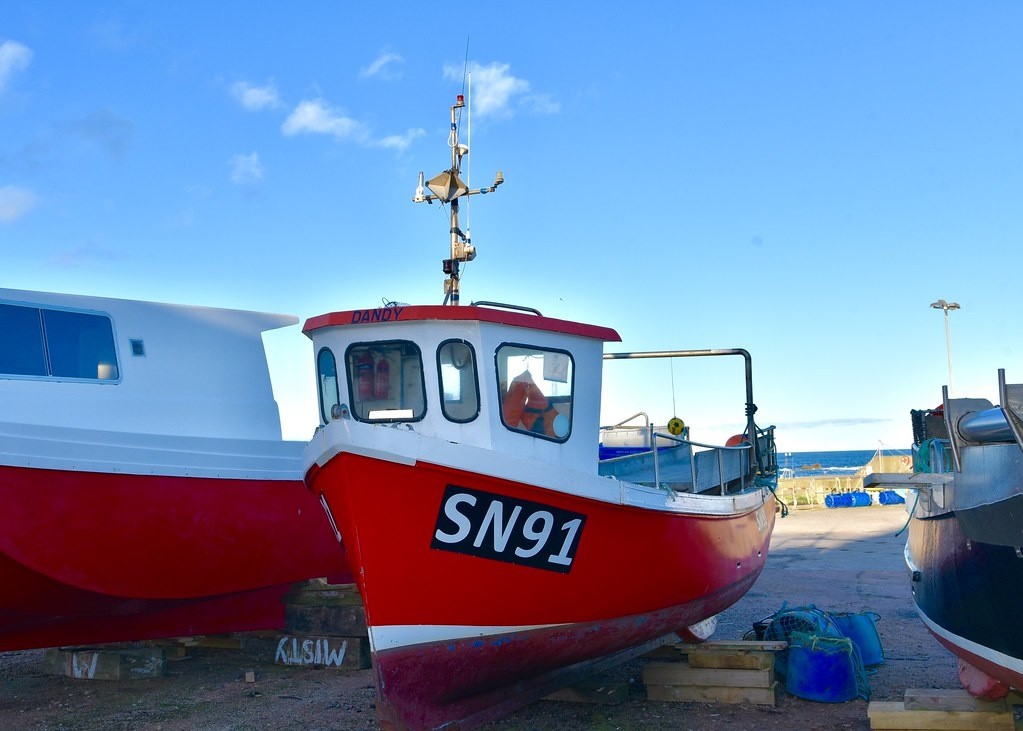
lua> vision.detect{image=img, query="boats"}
[860,365,1023,695]
[303,44,790,731]
[0,289,362,653]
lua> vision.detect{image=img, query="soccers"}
[667,418,685,436]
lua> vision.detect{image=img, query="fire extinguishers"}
[358,353,374,400]
[376,352,396,399]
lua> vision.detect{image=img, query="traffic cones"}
[931,300,962,398]
